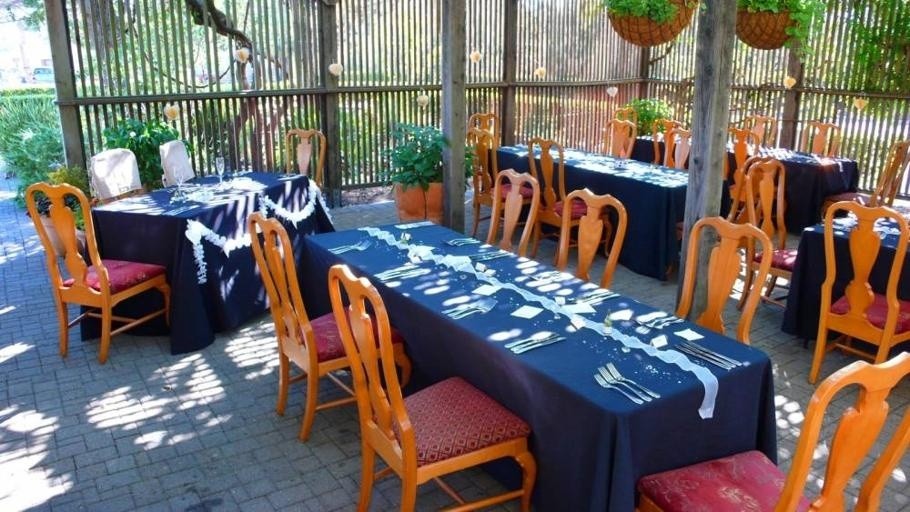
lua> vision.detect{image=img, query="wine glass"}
[216,157,226,189]
[172,160,188,203]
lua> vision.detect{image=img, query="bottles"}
[400,231,407,249]
[603,310,612,334]
[614,156,629,170]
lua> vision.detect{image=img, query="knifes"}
[673,339,742,372]
[394,221,435,229]
[466,250,514,263]
[380,266,424,281]
[504,333,567,355]
[565,290,620,306]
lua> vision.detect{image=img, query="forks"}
[327,240,372,255]
[593,362,662,404]
[632,315,686,330]
[441,236,480,247]
[525,271,562,281]
[441,297,498,321]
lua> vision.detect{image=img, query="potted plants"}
[736,2,818,66]
[598,0,699,46]
